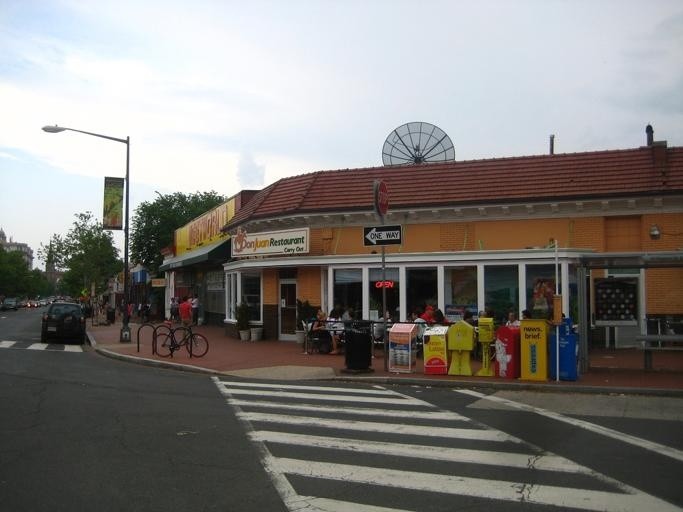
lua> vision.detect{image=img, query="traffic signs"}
[362,223,403,247]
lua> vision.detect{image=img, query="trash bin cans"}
[107,308,115,324]
[343,320,376,373]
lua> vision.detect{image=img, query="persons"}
[373,306,533,362]
[179,296,193,345]
[307,307,354,355]
[169,294,200,326]
[92,298,152,323]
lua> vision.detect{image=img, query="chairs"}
[301,316,357,355]
[371,319,428,359]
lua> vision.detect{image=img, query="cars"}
[0,295,72,312]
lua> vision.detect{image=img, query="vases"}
[251,328,264,342]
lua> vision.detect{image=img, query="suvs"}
[41,302,87,343]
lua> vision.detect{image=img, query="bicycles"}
[152,321,210,358]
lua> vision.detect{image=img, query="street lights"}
[38,123,135,344]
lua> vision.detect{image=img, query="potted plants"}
[235,304,251,341]
